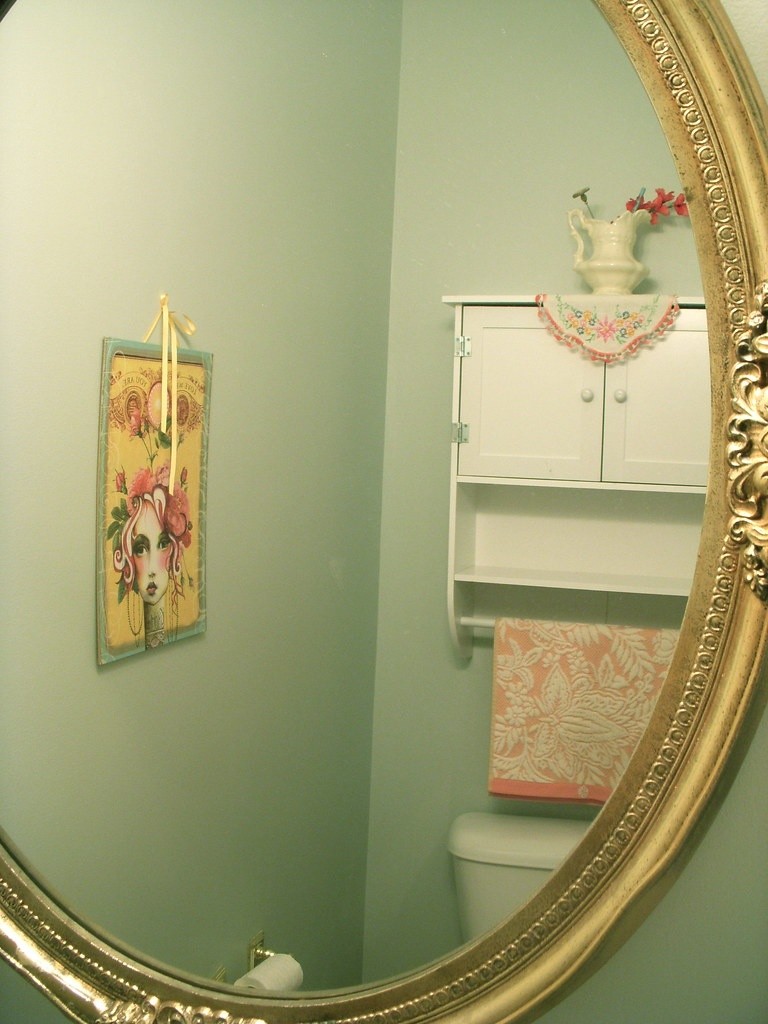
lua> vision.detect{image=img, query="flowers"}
[573,188,690,229]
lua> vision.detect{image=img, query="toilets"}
[445,811,594,947]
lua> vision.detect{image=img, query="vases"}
[566,208,653,297]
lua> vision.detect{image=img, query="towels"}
[487,616,682,807]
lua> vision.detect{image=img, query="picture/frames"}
[94,335,214,665]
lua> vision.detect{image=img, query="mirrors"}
[0,0,768,1024]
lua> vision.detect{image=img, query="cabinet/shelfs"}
[441,296,711,660]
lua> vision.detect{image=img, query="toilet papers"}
[233,953,304,992]
